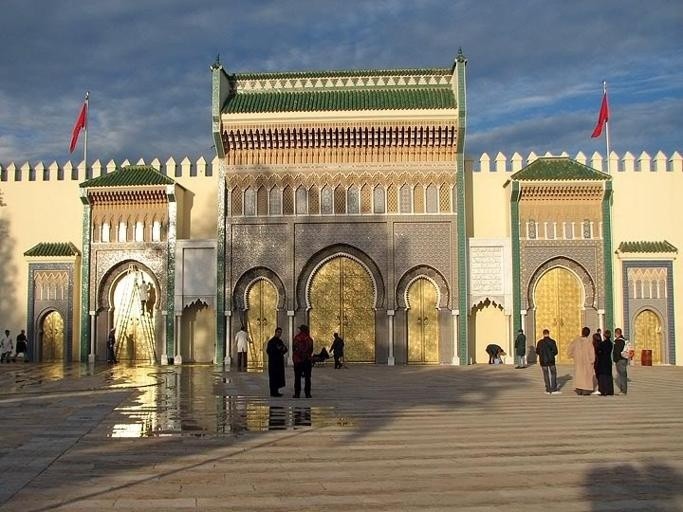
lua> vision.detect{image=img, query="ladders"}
[114,264,153,364]
[248,321,258,365]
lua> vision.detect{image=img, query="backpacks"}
[618,338,634,359]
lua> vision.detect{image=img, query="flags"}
[589,78,609,139]
[67,90,89,155]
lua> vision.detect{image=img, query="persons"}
[289,324,314,398]
[328,331,345,369]
[513,329,528,370]
[312,346,329,366]
[12,328,28,362]
[0,329,14,363]
[485,343,506,365]
[232,325,252,372]
[105,328,118,365]
[265,327,288,398]
[534,326,630,397]
